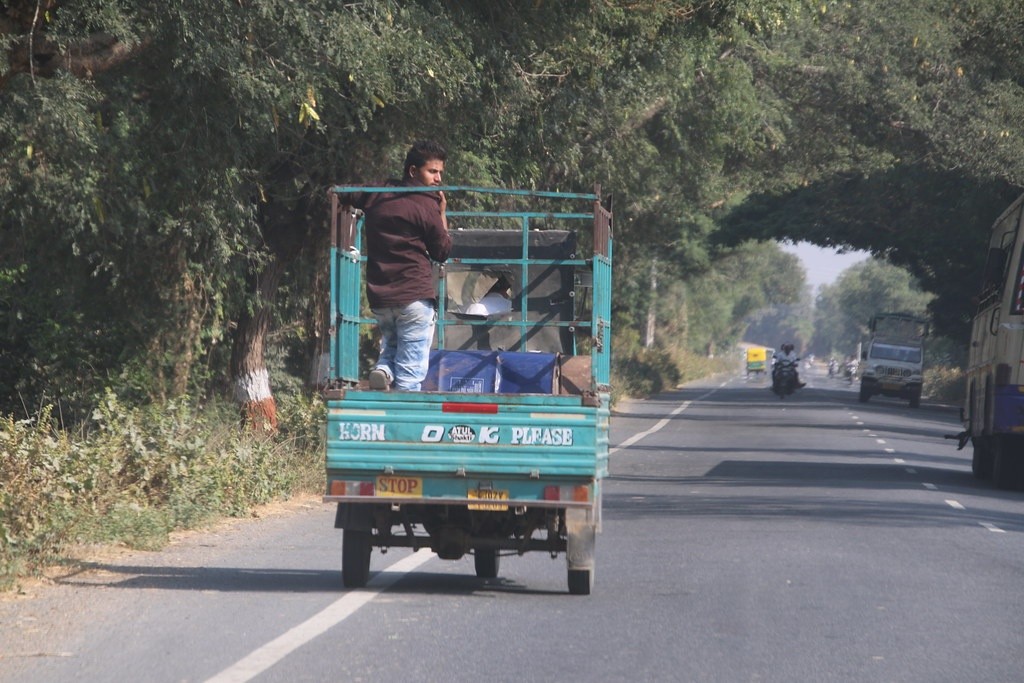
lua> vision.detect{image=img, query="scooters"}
[830,361,840,379]
[846,362,858,385]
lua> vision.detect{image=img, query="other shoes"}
[369,370,389,391]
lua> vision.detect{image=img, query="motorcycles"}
[745,346,770,375]
[772,355,801,398]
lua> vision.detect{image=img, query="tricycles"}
[326,182,614,593]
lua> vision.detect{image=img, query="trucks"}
[858,315,930,409]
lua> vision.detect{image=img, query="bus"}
[943,194,1024,491]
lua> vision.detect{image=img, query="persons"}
[465,273,514,316]
[846,355,860,381]
[771,342,805,389]
[326,141,452,393]
